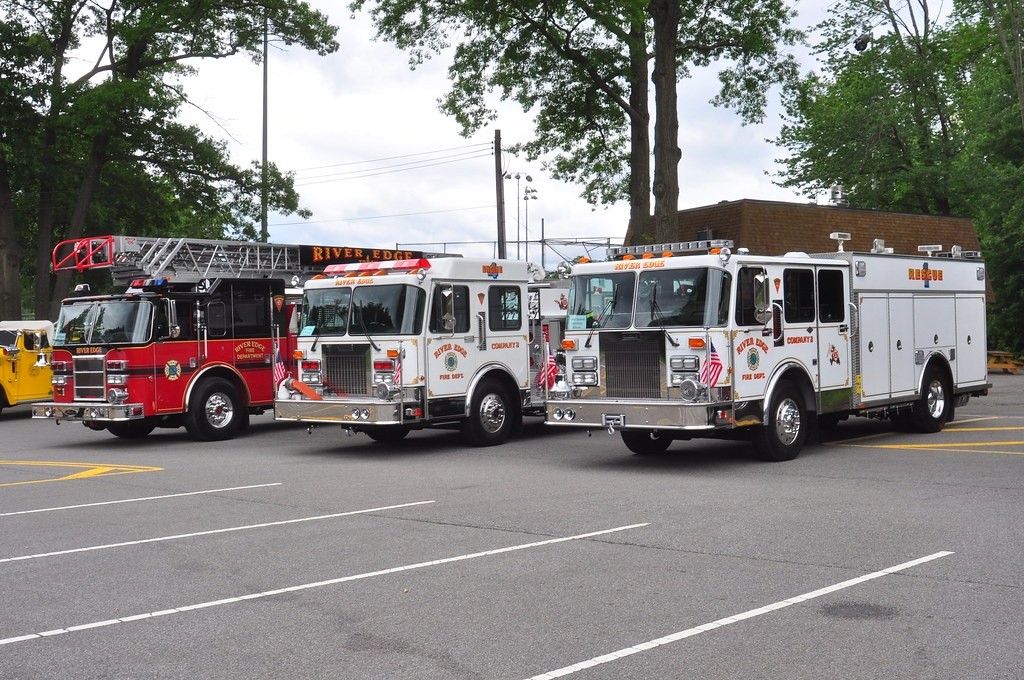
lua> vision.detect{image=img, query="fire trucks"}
[274,256,614,448]
[543,232,993,462]
[30,235,466,443]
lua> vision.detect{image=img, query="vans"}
[0,319,80,414]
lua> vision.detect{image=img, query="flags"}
[393,359,401,385]
[273,351,286,382]
[699,342,722,389]
[540,347,558,391]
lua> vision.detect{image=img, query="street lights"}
[504,170,532,260]
[524,186,538,261]
